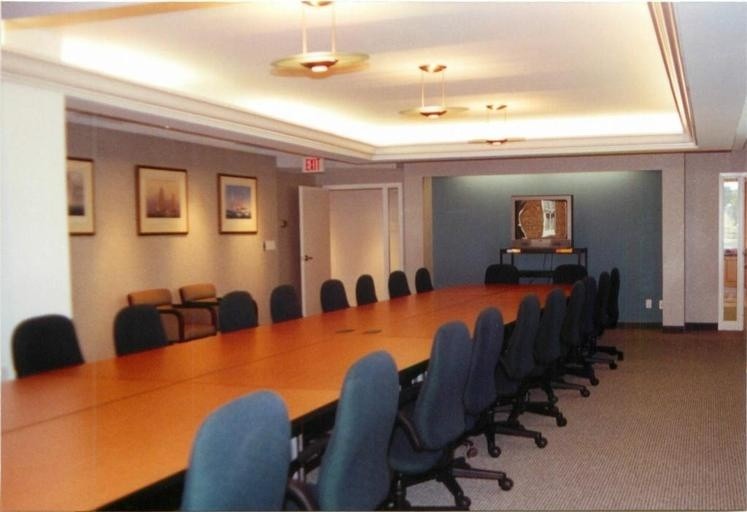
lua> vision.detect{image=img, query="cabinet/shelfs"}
[500,247,588,283]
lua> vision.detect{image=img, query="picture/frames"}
[135,165,189,236]
[66,157,95,235]
[218,172,259,234]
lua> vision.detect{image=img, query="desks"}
[1,284,578,511]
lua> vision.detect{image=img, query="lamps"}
[272,1,370,74]
[469,106,526,146]
[400,63,470,119]
